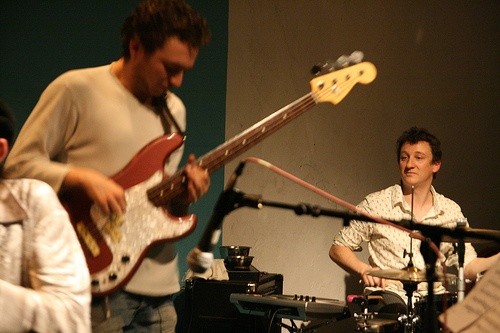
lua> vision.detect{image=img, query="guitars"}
[57,51,377,289]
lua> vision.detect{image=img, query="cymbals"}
[365,263,461,285]
[413,225,499,244]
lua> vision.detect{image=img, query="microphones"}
[186,160,245,273]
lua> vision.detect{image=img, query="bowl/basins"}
[227,245,251,256]
[227,255,255,268]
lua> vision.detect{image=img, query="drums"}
[356,315,399,333]
[410,291,463,333]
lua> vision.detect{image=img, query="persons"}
[0,0,210,333]
[329,126,500,309]
